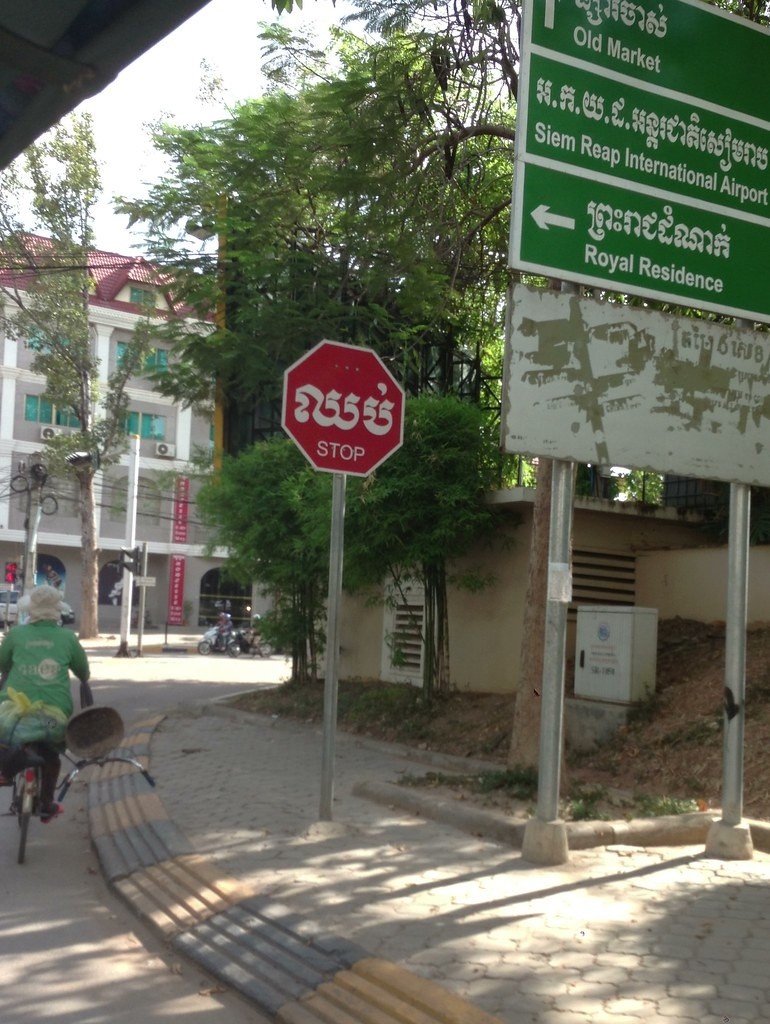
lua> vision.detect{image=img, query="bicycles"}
[55,680,156,802]
[2,747,57,864]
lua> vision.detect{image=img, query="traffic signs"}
[516,42,769,221]
[508,156,769,324]
[522,0,770,125]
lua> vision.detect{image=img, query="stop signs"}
[281,337,406,478]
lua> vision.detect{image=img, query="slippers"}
[40,801,64,823]
[1,775,10,784]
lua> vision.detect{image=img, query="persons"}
[250,614,265,658]
[0,586,90,824]
[215,612,233,651]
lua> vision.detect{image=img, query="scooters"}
[197,622,243,658]
[232,627,276,659]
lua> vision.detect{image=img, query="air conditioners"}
[155,442,176,458]
[40,426,62,441]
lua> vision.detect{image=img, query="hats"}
[18,584,71,617]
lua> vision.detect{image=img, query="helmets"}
[253,614,260,621]
[223,614,231,621]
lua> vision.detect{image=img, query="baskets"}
[64,706,125,758]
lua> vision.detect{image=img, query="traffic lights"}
[120,546,141,576]
[4,561,17,583]
